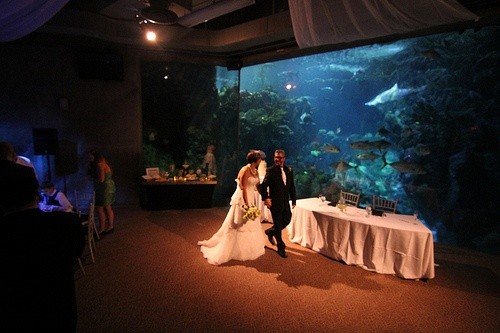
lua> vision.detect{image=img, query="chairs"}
[84,203,96,264]
[340,189,360,207]
[373,195,399,214]
[73,190,101,241]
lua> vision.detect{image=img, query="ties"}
[281,167,287,186]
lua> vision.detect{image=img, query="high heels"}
[105,227,114,233]
[99,231,106,236]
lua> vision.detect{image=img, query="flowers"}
[241,205,260,221]
[289,167,291,171]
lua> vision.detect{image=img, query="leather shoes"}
[265,230,275,245]
[278,251,288,258]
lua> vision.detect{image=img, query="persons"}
[0,143,116,333]
[198,149,296,265]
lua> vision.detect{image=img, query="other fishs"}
[320,138,433,190]
[364,83,412,107]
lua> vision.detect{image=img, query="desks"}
[142,179,217,210]
[36,208,86,277]
[287,197,435,279]
